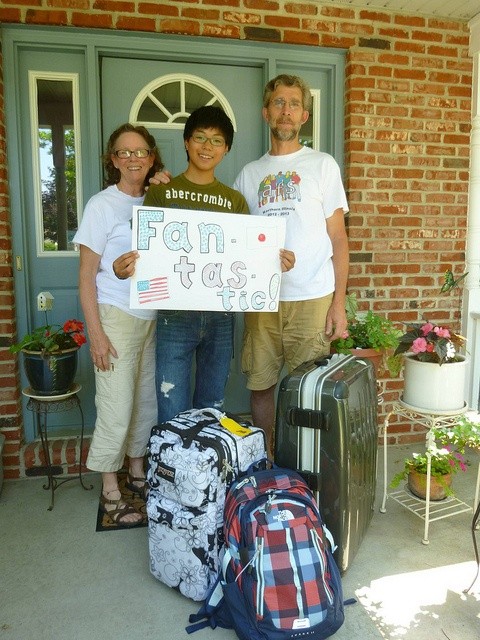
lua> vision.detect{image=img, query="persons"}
[71,122,158,528]
[231,74,349,463]
[112,105,296,426]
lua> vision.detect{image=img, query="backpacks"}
[185,458,356,639]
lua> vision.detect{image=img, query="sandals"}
[124,471,147,501]
[99,490,143,527]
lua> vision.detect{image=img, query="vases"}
[403,465,453,504]
[20,341,82,401]
[397,352,470,416]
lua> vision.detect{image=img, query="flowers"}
[8,291,88,358]
[388,418,480,497]
[393,269,470,365]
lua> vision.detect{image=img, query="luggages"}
[274,353,379,578]
[146,407,268,603]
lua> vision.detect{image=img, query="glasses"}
[114,149,152,159]
[189,133,225,146]
[268,97,304,111]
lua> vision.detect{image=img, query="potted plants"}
[334,293,398,379]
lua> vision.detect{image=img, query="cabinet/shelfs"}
[378,408,479,545]
[26,394,94,512]
